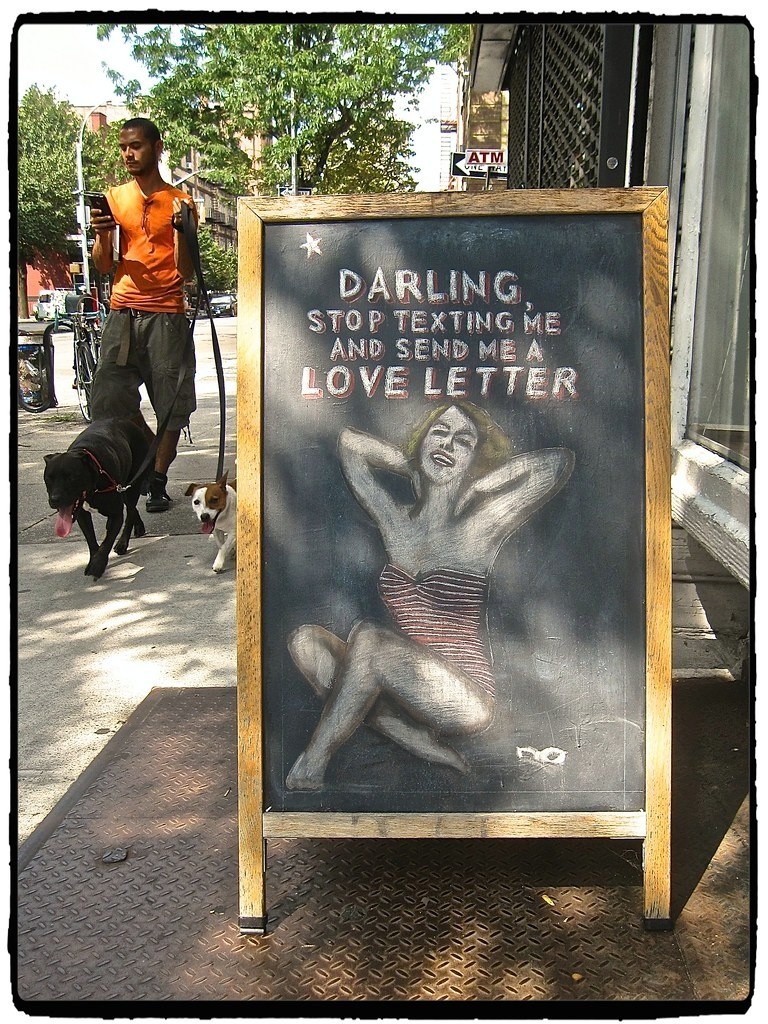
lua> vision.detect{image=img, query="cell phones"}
[86,189,116,230]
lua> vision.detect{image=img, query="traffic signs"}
[450,151,510,179]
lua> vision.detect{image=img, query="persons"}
[89,117,199,514]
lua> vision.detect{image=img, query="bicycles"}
[51,306,105,420]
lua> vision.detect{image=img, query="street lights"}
[77,102,108,292]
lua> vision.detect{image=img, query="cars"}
[208,294,237,318]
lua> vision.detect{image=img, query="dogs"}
[43,416,152,581]
[184,470,237,572]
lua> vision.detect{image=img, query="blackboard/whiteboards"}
[226,186,686,841]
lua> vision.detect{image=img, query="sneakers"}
[146,471,170,512]
